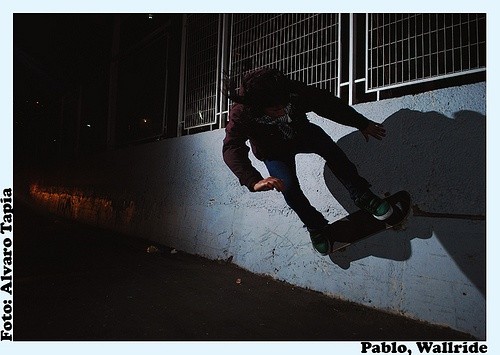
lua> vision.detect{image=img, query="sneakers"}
[309,227,331,254]
[358,193,393,219]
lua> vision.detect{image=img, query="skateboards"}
[312,189,411,256]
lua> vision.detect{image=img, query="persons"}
[222,67,394,256]
